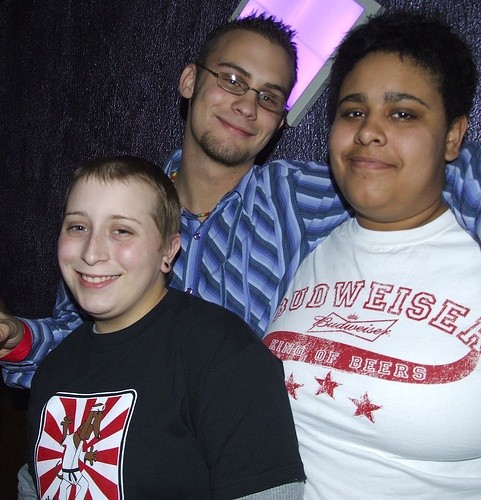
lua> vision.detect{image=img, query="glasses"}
[196,60,289,113]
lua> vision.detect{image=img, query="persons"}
[261,11,479,499]
[0,11,481,396]
[16,154,307,500]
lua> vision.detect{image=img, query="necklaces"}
[170,169,214,219]
